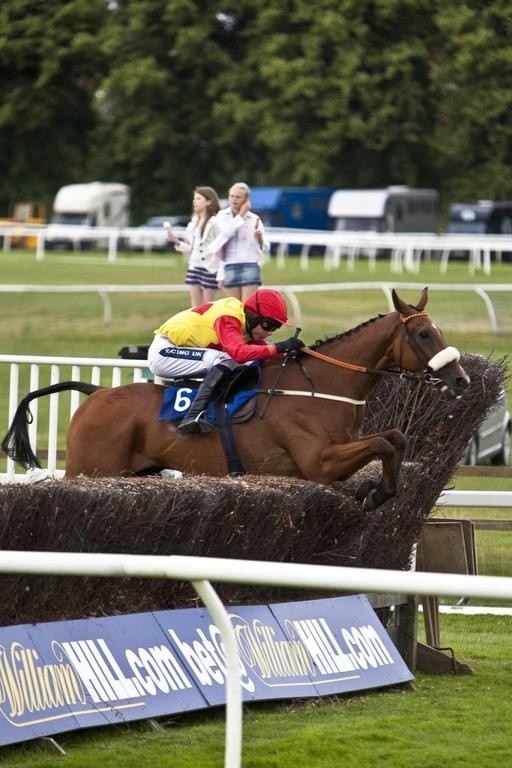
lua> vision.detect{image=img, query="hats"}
[244,289,294,328]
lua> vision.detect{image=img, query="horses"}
[1,285,474,513]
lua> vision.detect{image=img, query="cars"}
[128,214,191,253]
[455,391,512,466]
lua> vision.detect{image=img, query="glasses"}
[260,322,278,331]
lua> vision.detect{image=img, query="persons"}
[164,186,219,304]
[204,185,269,304]
[146,286,305,437]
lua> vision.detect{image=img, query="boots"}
[178,365,233,433]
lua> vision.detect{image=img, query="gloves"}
[274,337,305,353]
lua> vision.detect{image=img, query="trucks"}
[246,184,339,255]
[326,184,441,262]
[438,198,512,261]
[43,179,133,252]
